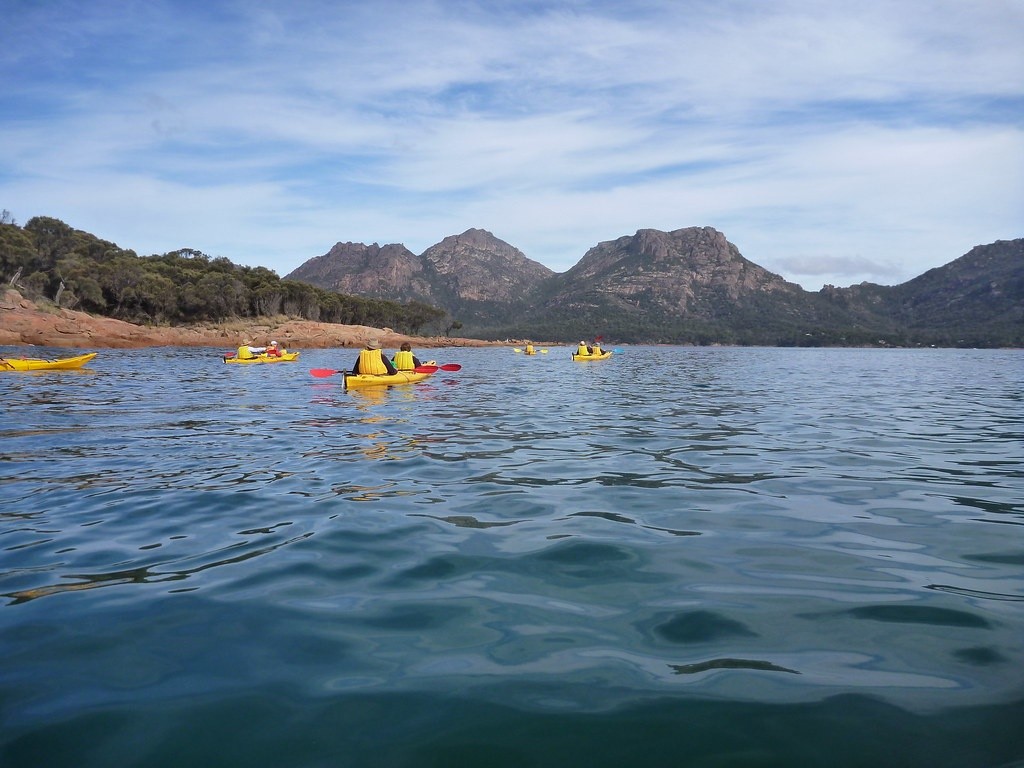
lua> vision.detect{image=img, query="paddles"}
[604,349,624,355]
[513,347,547,354]
[309,363,462,380]
[223,350,278,354]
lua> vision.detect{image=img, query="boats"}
[524,351,536,355]
[223,352,300,364]
[0,352,98,371]
[342,360,437,388]
[572,351,613,361]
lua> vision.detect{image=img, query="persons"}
[577,341,593,356]
[524,341,535,354]
[237,339,267,359]
[352,337,398,375]
[591,342,606,356]
[391,343,421,372]
[265,340,281,357]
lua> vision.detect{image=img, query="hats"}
[364,338,382,349]
[594,342,600,346]
[528,341,532,344]
[580,341,585,345]
[271,341,277,344]
[241,339,252,345]
